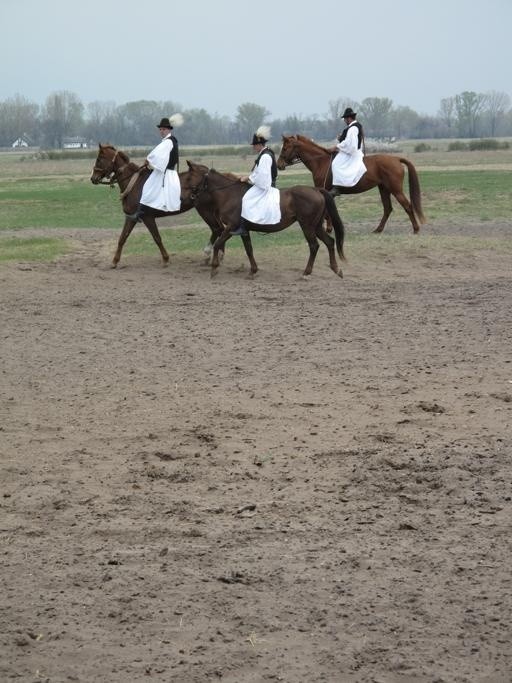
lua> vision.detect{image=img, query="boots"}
[229,218,248,236]
[125,208,145,223]
[328,185,341,196]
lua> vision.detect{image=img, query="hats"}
[250,134,268,145]
[157,117,173,129]
[341,108,358,117]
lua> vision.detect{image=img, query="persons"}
[326,114,368,188]
[239,141,282,226]
[138,126,182,212]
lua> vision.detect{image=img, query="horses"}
[90,142,238,269]
[276,132,426,234]
[185,159,347,282]
